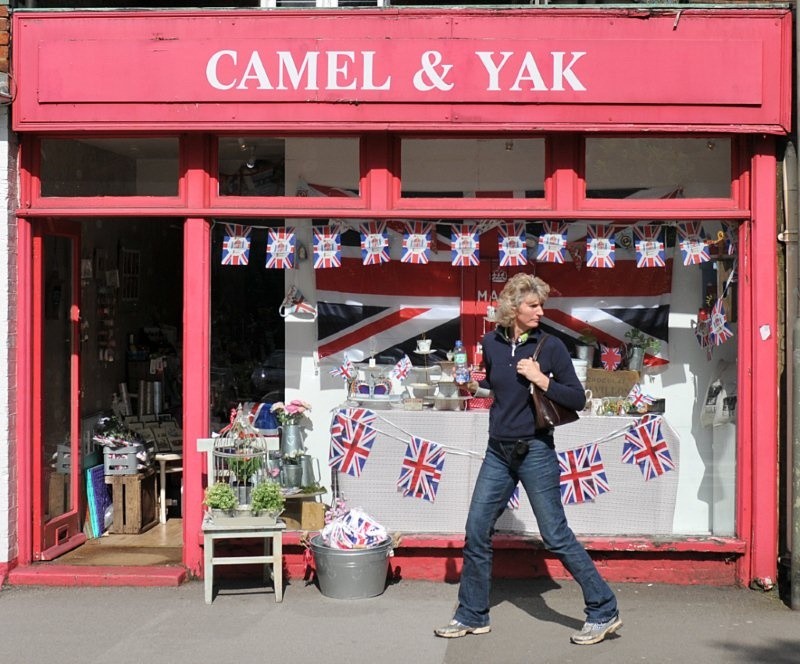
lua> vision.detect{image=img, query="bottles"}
[454,340,469,383]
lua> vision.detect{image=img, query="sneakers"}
[571,610,623,644]
[434,618,490,638]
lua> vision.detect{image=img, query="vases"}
[276,424,304,487]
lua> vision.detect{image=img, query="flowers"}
[284,446,306,466]
[269,400,311,426]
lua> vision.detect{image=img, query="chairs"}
[196,437,287,606]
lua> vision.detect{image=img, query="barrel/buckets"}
[309,534,394,599]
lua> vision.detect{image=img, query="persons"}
[252,328,286,396]
[434,273,623,644]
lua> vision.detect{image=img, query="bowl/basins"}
[416,339,432,352]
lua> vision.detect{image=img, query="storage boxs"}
[104,441,157,534]
[48,443,71,520]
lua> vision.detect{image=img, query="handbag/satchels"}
[529,334,580,429]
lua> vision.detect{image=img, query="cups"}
[120,381,162,414]
[591,398,610,416]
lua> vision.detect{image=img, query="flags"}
[220,218,738,384]
[328,407,674,510]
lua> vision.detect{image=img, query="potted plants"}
[625,327,661,370]
[575,319,597,369]
[203,457,285,517]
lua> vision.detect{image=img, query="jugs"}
[265,425,320,488]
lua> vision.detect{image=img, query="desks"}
[331,400,681,536]
[153,453,183,524]
